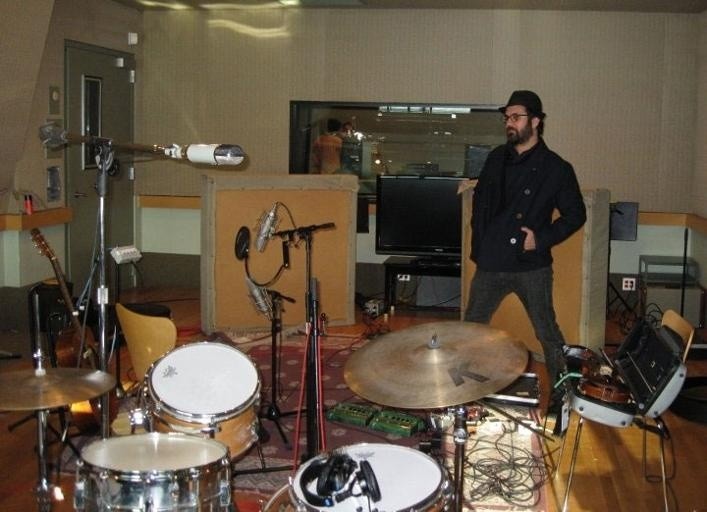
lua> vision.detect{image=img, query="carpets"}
[47,333,559,511]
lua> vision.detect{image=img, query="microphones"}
[254,210,278,253]
[164,141,244,169]
[244,276,274,317]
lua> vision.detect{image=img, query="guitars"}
[29,228,115,432]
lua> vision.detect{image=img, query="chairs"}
[554,307,697,511]
[114,303,180,435]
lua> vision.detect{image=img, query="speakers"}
[609,202,640,241]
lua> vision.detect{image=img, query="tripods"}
[7,333,91,460]
[255,291,308,449]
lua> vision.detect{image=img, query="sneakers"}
[547,401,564,414]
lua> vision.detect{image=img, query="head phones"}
[291,449,381,512]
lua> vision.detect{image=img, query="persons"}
[337,122,353,139]
[309,117,342,174]
[462,90,587,438]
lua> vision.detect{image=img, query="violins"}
[580,352,630,403]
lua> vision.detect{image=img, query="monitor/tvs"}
[375,173,471,264]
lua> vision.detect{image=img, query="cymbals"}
[344,321,529,409]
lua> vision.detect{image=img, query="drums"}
[142,341,263,461]
[287,443,456,512]
[0,367,116,412]
[72,431,232,512]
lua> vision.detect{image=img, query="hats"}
[498,91,545,119]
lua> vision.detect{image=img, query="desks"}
[379,256,462,318]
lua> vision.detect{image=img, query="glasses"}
[502,114,527,124]
